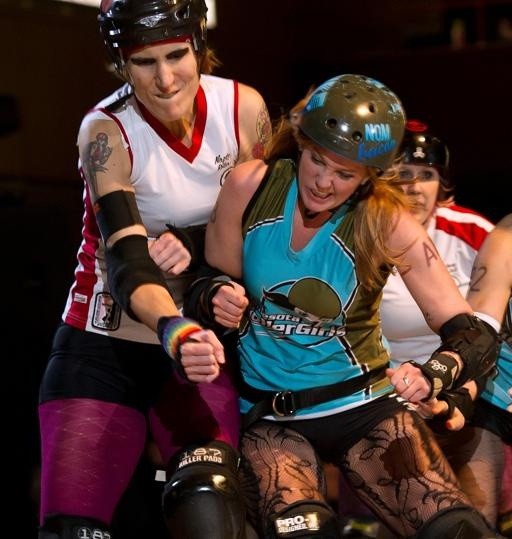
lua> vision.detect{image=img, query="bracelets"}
[155,316,199,359]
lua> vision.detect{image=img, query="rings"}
[402,376,410,387]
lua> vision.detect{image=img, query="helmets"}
[95,0,209,80]
[392,120,452,183]
[295,72,408,178]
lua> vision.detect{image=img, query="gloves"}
[399,353,477,434]
[153,274,235,388]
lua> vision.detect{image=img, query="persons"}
[37,1,272,537]
[183,74,503,536]
[321,117,498,537]
[416,217,512,538]
